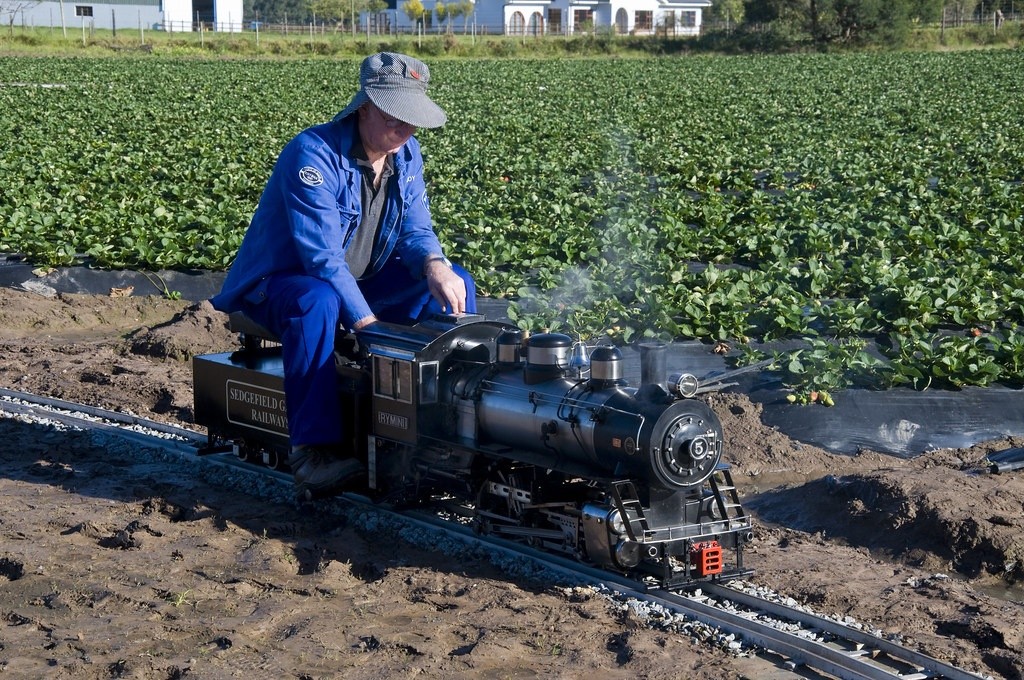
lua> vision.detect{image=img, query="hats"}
[360,52,448,128]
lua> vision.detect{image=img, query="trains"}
[186,308,758,594]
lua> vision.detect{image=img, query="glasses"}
[373,103,403,128]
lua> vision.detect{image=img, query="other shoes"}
[287,445,365,499]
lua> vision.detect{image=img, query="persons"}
[207,49,479,488]
